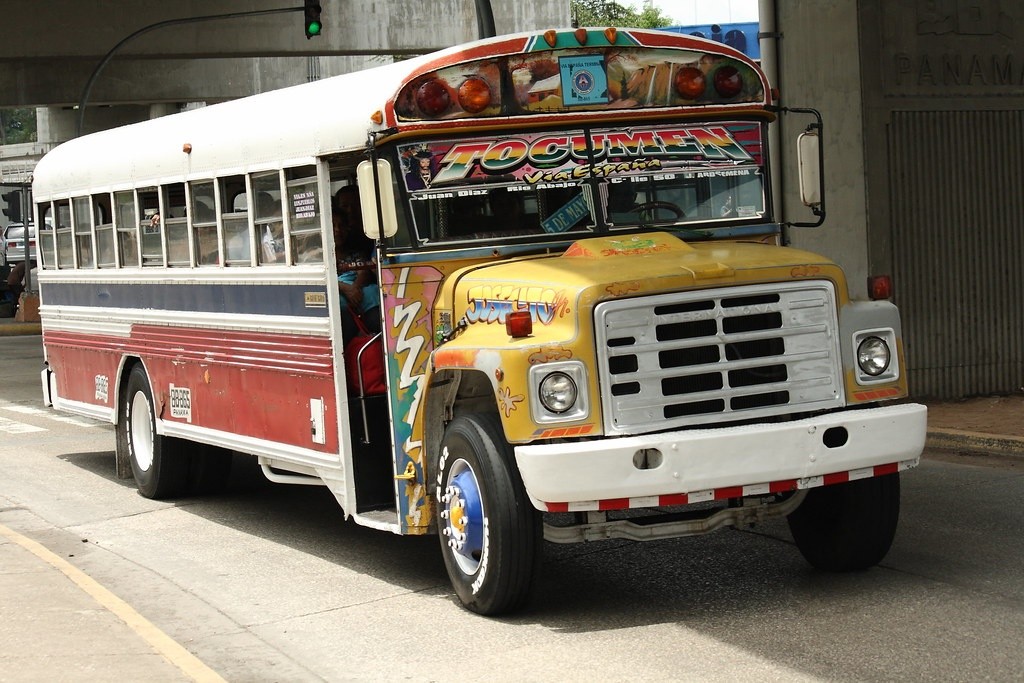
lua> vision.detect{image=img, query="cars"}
[4,223,53,267]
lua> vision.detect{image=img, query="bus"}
[31,29,928,621]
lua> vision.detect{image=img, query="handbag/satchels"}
[345,335,387,394]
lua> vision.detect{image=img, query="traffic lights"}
[305,0,322,40]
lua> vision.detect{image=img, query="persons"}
[42,191,322,268]
[479,192,540,230]
[0,226,8,305]
[7,260,37,318]
[333,184,379,348]
